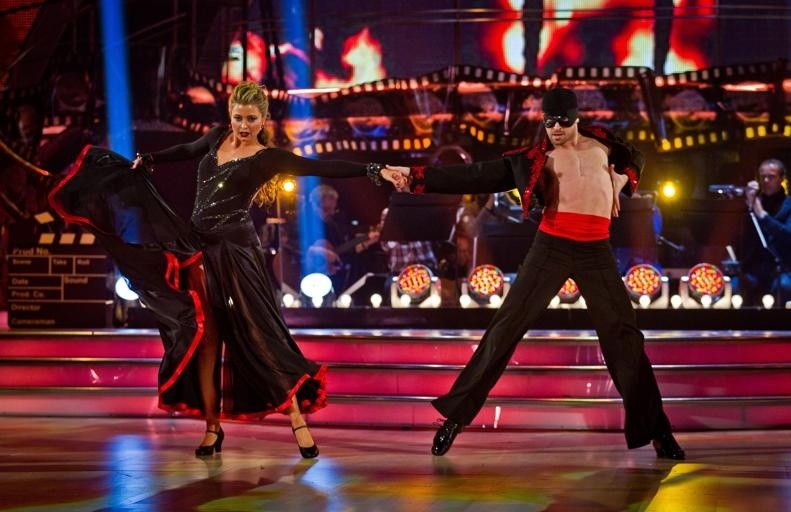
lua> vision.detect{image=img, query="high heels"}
[195,426,224,458]
[290,424,320,460]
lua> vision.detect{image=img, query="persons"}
[464,185,523,273]
[744,158,790,306]
[45,82,409,459]
[384,89,688,459]
[289,183,381,306]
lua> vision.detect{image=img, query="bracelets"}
[361,240,368,250]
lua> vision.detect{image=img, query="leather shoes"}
[652,441,685,460]
[431,418,466,457]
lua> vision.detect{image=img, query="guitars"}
[268,224,383,291]
